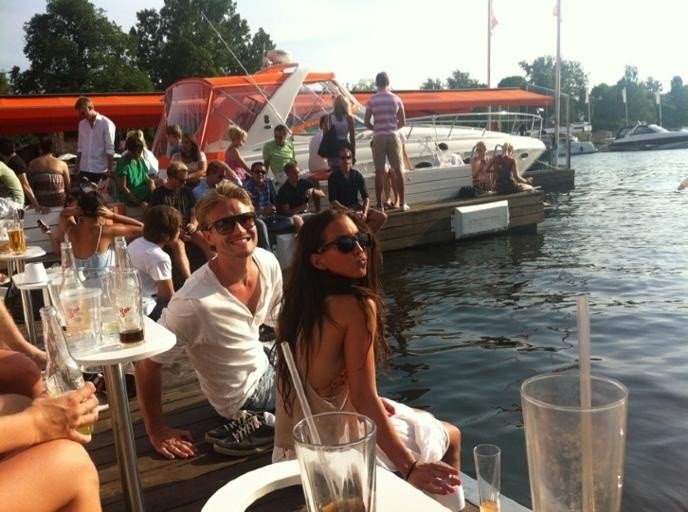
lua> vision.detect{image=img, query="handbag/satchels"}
[318,114,336,158]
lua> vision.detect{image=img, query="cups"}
[518,372,631,512]
[5,221,26,256]
[59,287,108,354]
[104,266,145,346]
[473,442,503,512]
[430,481,463,512]
[290,410,378,512]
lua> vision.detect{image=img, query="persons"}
[472,142,498,198]
[0,71,466,511]
[485,143,551,207]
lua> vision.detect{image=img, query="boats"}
[138,54,550,206]
[606,121,688,152]
[531,132,599,157]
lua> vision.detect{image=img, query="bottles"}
[36,304,95,437]
[11,208,21,225]
[55,241,90,348]
[111,236,134,272]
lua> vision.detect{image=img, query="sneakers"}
[400,204,410,212]
[213,414,275,457]
[205,406,275,444]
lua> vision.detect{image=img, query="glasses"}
[341,156,351,160]
[254,169,266,175]
[317,232,371,255]
[205,212,258,234]
